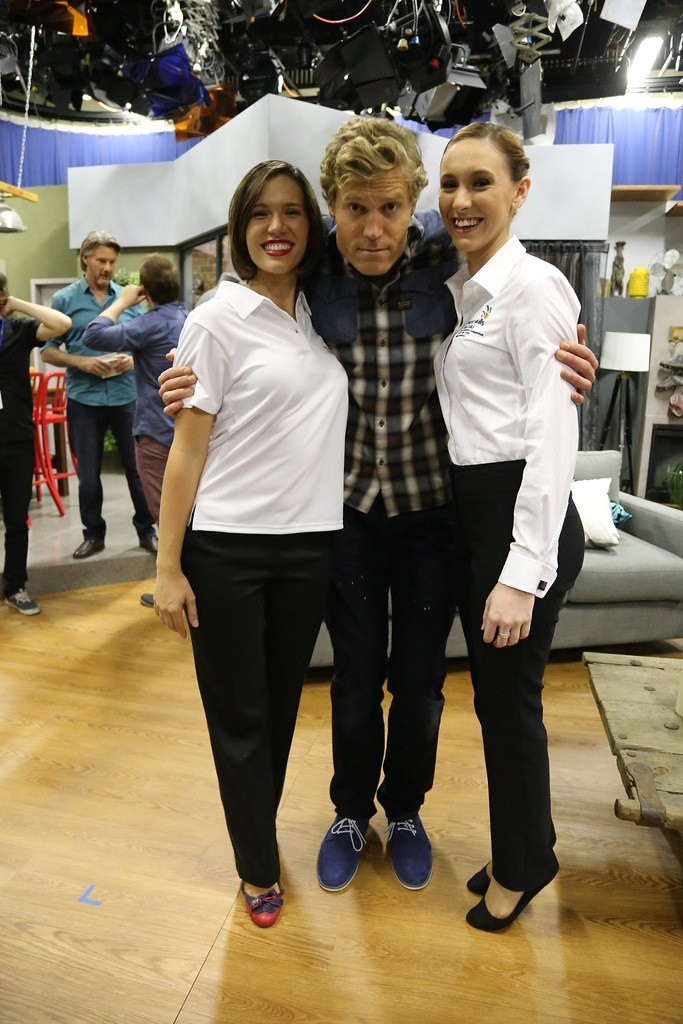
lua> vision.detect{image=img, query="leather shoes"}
[73,537,105,559]
[139,536,158,555]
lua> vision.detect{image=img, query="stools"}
[26,372,79,526]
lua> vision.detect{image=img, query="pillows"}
[570,477,632,550]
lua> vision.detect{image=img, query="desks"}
[32,387,72,496]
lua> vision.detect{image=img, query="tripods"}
[598,371,634,496]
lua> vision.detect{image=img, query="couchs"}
[310,450,683,666]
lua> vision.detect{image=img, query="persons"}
[0,272,73,615]
[82,256,191,608]
[433,120,586,933]
[40,229,158,559]
[300,116,463,893]
[154,160,351,928]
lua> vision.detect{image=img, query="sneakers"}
[316,815,370,892]
[4,589,40,615]
[385,815,432,890]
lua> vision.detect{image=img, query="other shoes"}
[240,881,285,928]
[465,850,560,931]
[140,593,153,608]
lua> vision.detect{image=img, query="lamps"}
[597,331,651,496]
[0,0,683,138]
[0,192,27,232]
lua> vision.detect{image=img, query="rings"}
[498,633,510,638]
